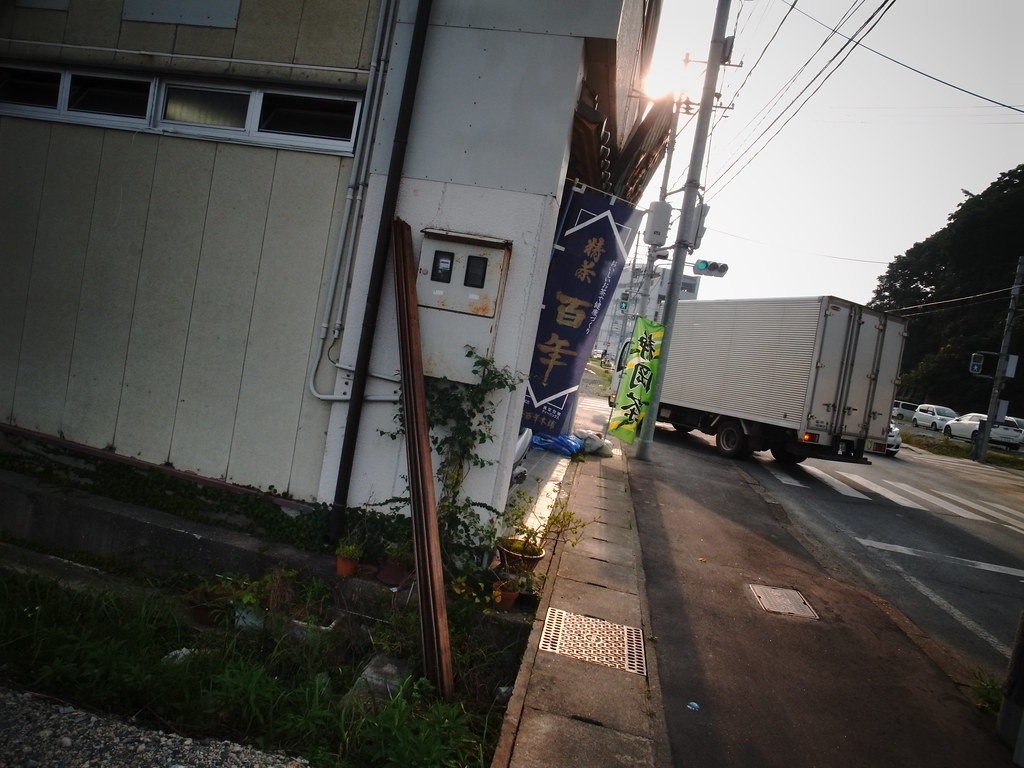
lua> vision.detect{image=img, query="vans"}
[892,400,920,420]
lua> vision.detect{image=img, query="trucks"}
[592,350,603,359]
[600,294,910,464]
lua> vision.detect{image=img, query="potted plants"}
[490,487,604,610]
[378,542,415,587]
[334,534,362,580]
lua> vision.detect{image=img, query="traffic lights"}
[694,259,728,277]
[970,353,985,374]
[619,292,629,310]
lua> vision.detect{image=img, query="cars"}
[943,413,1023,451]
[887,419,902,455]
[912,403,958,431]
